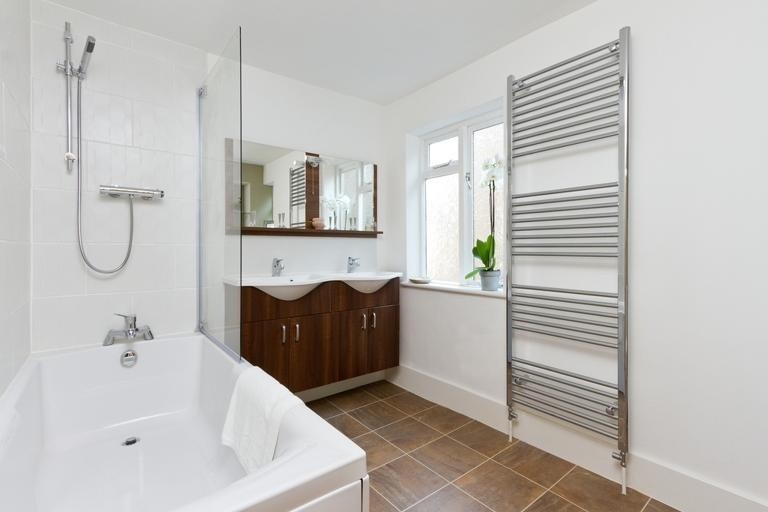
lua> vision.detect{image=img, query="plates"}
[409,279,431,284]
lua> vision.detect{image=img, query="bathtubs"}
[1,328,369,511]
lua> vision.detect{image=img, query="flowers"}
[465,154,503,280]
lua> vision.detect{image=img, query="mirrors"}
[226,138,381,238]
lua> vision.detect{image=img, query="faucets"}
[347,254,361,273]
[103,309,154,345]
[271,257,285,276]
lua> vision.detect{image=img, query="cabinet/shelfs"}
[224,277,401,405]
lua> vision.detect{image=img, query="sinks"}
[260,276,313,285]
[350,271,388,280]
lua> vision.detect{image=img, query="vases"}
[481,271,500,292]
